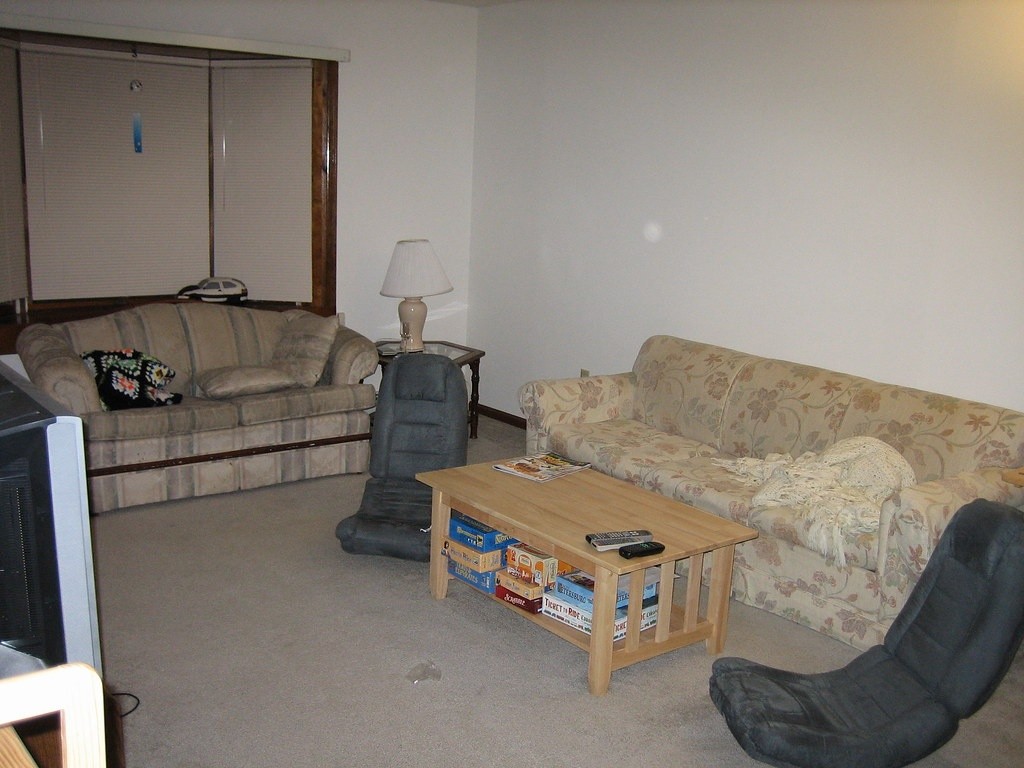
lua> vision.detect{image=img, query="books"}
[492,451,592,483]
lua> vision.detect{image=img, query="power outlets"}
[581,369,590,378]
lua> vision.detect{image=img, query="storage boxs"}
[441,535,508,574]
[495,586,542,615]
[555,571,656,613]
[450,515,519,554]
[496,568,555,600]
[542,590,658,643]
[507,542,581,587]
[447,557,502,594]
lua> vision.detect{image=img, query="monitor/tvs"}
[0,359,103,682]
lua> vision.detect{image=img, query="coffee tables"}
[415,454,759,697]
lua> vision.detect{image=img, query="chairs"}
[709,499,1024,768]
[335,354,468,563]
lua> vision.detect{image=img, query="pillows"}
[193,365,297,400]
[96,366,183,412]
[77,348,175,389]
[267,312,346,387]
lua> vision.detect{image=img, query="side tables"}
[359,341,485,439]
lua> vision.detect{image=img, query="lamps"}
[380,239,453,352]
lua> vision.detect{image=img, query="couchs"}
[517,335,1024,653]
[16,302,379,517]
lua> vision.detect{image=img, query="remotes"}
[592,537,644,552]
[619,542,665,559]
[585,530,653,545]
